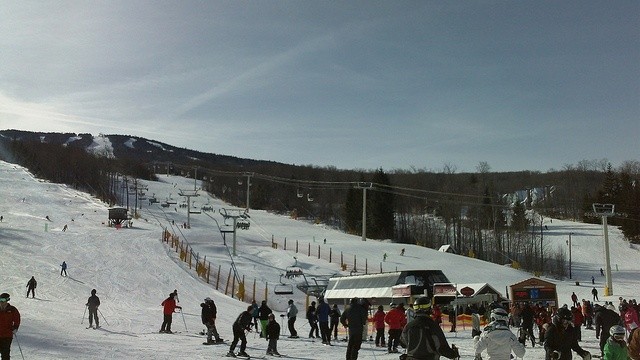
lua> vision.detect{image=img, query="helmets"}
[490,308,508,326]
[204,298,210,301]
[268,313,274,317]
[0,293,10,302]
[413,297,432,313]
[390,302,397,308]
[556,307,574,329]
[349,298,357,305]
[318,296,324,303]
[610,325,625,343]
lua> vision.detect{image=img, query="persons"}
[600,296,640,360]
[591,276,595,285]
[581,299,600,360]
[448,305,457,332]
[265,313,280,356]
[200,299,223,344]
[159,293,182,333]
[405,304,414,323]
[384,302,405,353]
[431,304,442,326]
[172,289,179,313]
[383,253,387,261]
[361,298,371,342]
[229,306,255,358]
[571,292,578,307]
[257,300,272,337]
[287,299,298,337]
[62,224,68,232]
[400,248,406,256]
[206,297,217,322]
[250,300,259,334]
[306,301,320,338]
[26,275,37,298]
[330,304,341,339]
[592,287,598,301]
[545,225,548,230]
[374,305,386,347]
[0,293,21,360]
[313,296,332,345]
[400,297,460,360]
[60,260,67,276]
[86,288,100,328]
[472,308,526,360]
[600,268,604,277]
[340,297,365,360]
[521,303,581,360]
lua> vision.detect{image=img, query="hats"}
[247,306,254,312]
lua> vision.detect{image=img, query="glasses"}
[0,298,6,302]
[612,334,625,342]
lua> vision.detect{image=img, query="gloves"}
[582,351,592,360]
[551,350,561,360]
[472,329,482,338]
[519,337,525,343]
[453,346,460,358]
[12,329,18,334]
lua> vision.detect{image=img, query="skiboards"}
[152,330,182,334]
[225,353,265,360]
[288,336,300,338]
[85,324,102,329]
[202,342,226,345]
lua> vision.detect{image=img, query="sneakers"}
[207,340,214,343]
[239,352,249,357]
[393,348,399,352]
[273,351,280,355]
[381,344,386,346]
[388,348,393,353]
[266,352,273,355]
[376,344,379,346]
[216,339,223,343]
[227,352,237,357]
